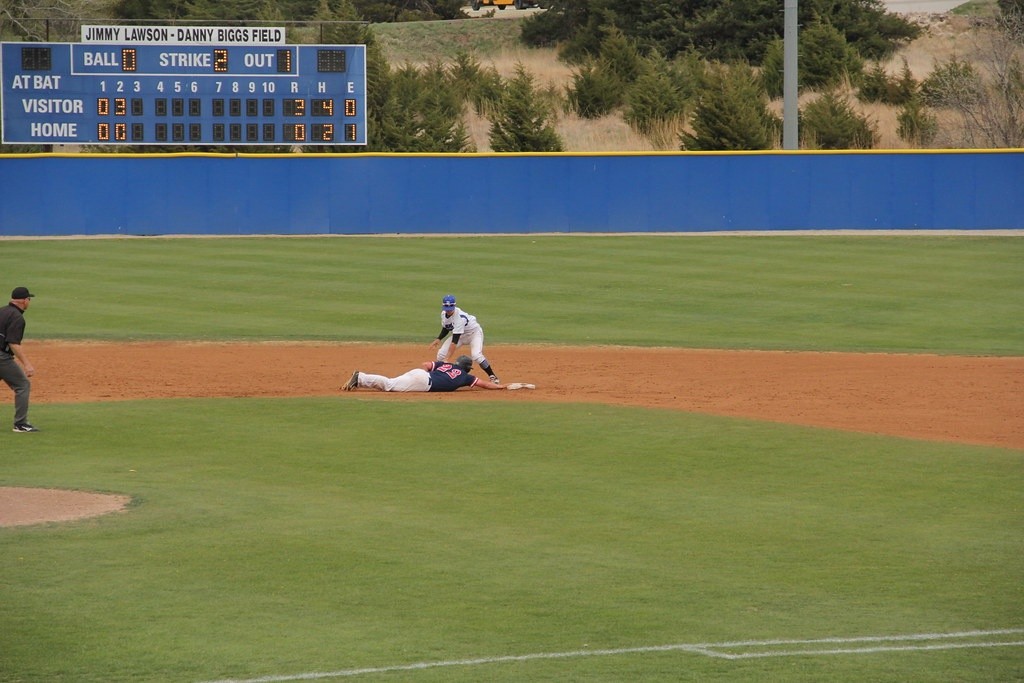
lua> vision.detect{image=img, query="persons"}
[0,287,40,433]
[428,296,502,384]
[338,354,514,394]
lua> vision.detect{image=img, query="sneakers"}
[490,375,499,384]
[13,423,43,432]
[340,369,359,391]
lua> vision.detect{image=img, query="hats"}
[12,287,35,299]
[442,295,456,312]
[457,355,474,369]
